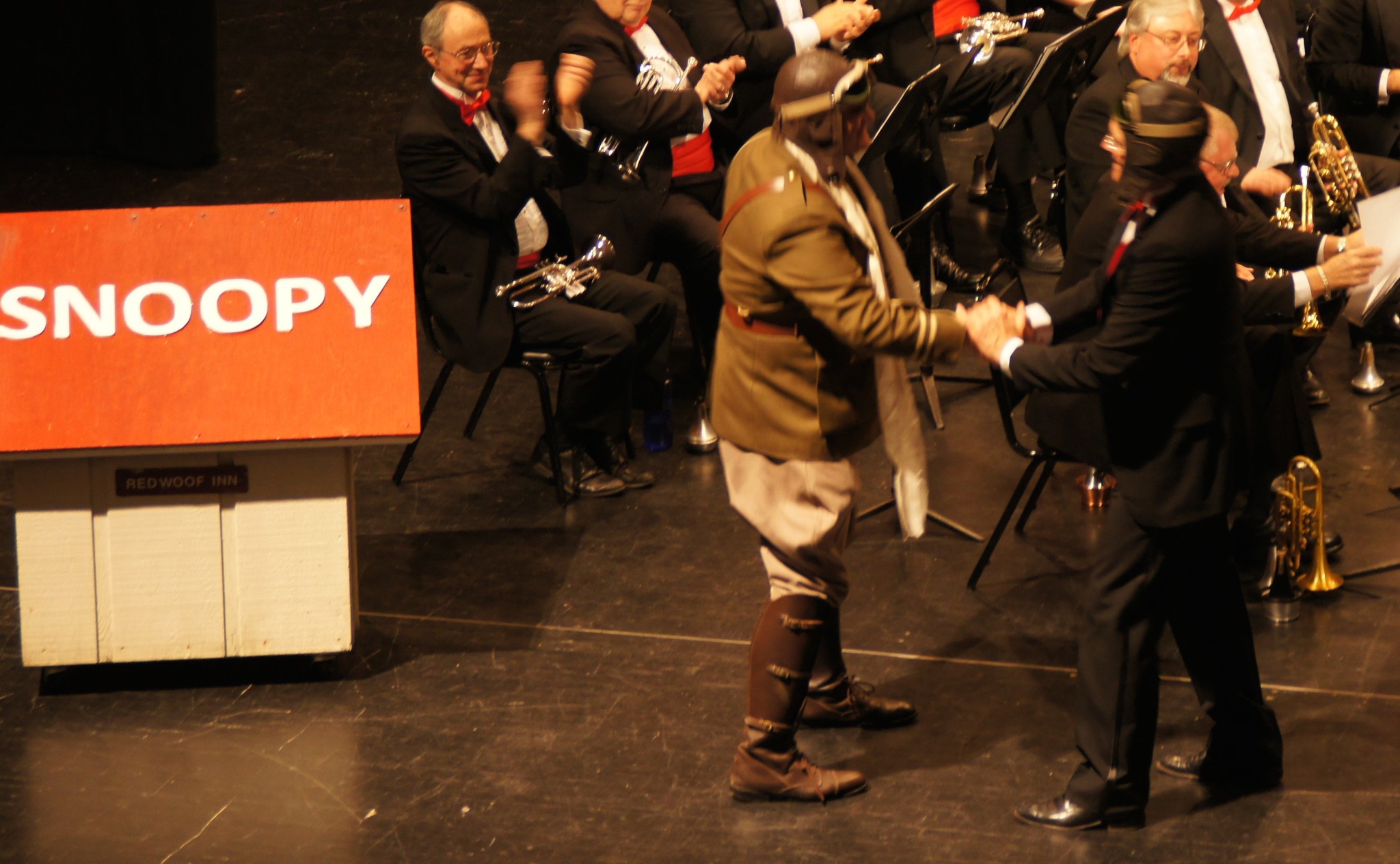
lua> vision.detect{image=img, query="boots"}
[730,590,868,802]
[803,605,919,729]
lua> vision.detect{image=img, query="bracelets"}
[1317,265,1334,302]
[1338,235,1348,253]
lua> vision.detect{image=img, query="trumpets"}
[1307,101,1371,229]
[598,55,700,185]
[493,235,617,311]
[1262,164,1324,338]
[1272,455,1345,592]
[951,5,1050,64]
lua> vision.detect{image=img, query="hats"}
[1117,77,1209,208]
[771,48,884,123]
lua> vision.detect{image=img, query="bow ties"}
[1228,0,1262,21]
[439,87,492,126]
[624,15,649,37]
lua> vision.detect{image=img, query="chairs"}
[968,258,1131,589]
[392,122,632,504]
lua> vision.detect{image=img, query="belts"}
[725,300,814,336]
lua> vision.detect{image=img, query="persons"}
[715,43,982,802]
[968,78,1291,834]
[528,1,1400,455]
[392,1,680,498]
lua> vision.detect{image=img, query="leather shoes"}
[530,435,627,497]
[684,400,718,453]
[1014,791,1147,833]
[1262,506,1345,557]
[586,441,655,488]
[1156,749,1284,788]
[1349,304,1400,352]
[932,247,988,292]
[1045,193,1067,228]
[1302,363,1330,406]
[1003,214,1065,272]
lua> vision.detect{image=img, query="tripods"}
[850,43,986,545]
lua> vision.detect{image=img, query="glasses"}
[425,40,501,65]
[1104,134,1126,155]
[1144,29,1206,53]
[1199,152,1239,174]
